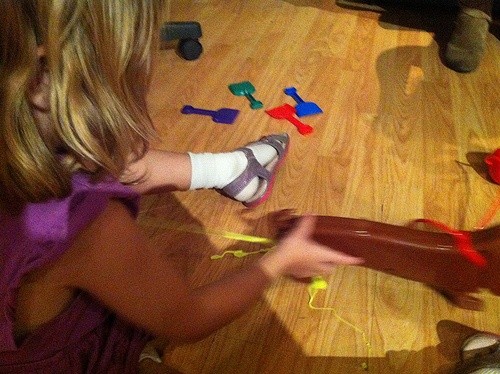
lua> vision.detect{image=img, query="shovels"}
[227,80,264,109]
[283,86,323,117]
[264,104,313,135]
[181,103,239,125]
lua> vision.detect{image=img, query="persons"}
[0,0,364,374]
[375,0,497,73]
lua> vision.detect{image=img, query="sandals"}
[221,130,290,207]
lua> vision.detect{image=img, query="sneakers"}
[439,6,492,75]
